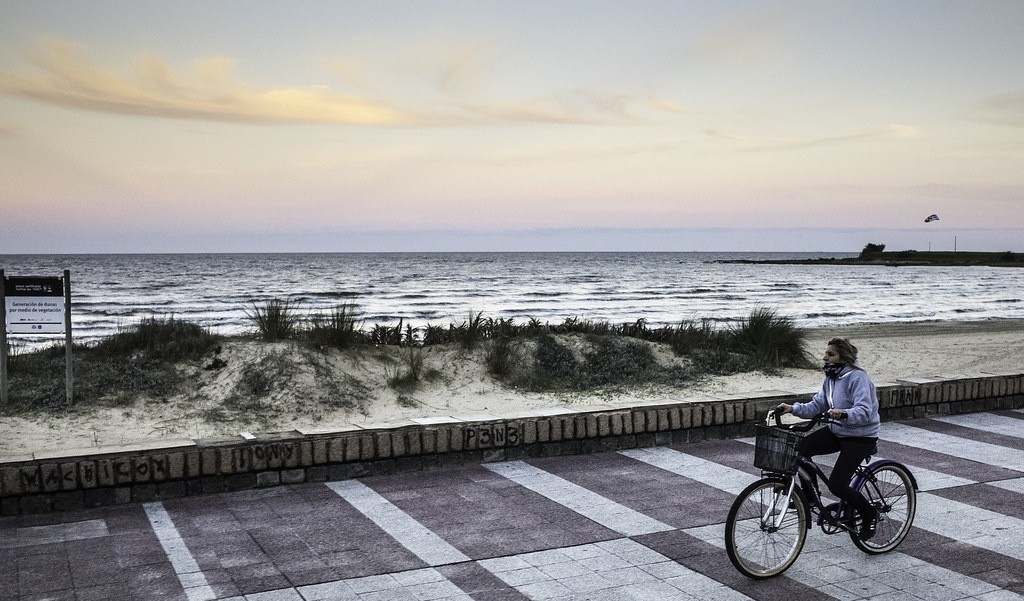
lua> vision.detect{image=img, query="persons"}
[777,337,881,540]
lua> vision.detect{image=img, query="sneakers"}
[789,491,821,508]
[860,506,880,541]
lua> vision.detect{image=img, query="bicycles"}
[724,402,919,581]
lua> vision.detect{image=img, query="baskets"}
[754,423,808,476]
